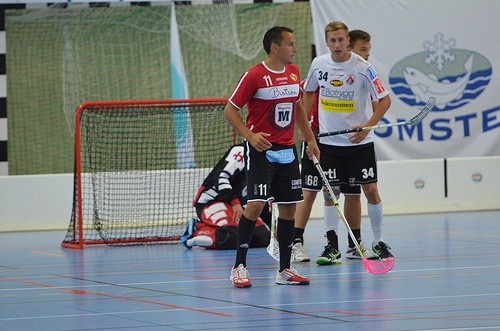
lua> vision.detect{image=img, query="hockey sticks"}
[306,146,394,274]
[315,97,436,139]
[267,202,294,263]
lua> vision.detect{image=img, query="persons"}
[181,142,272,249]
[293,30,379,262]
[223,26,320,287]
[298,21,395,265]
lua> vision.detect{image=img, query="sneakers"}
[372,240,395,261]
[315,244,342,265]
[346,244,374,259]
[230,263,253,287]
[275,267,310,285]
[292,242,311,262]
